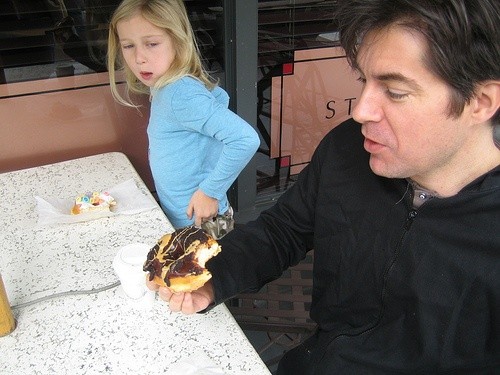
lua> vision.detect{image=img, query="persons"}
[107,0,261,240]
[145,0,500,374]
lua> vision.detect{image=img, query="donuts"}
[70,188,118,216]
[141,226,221,292]
